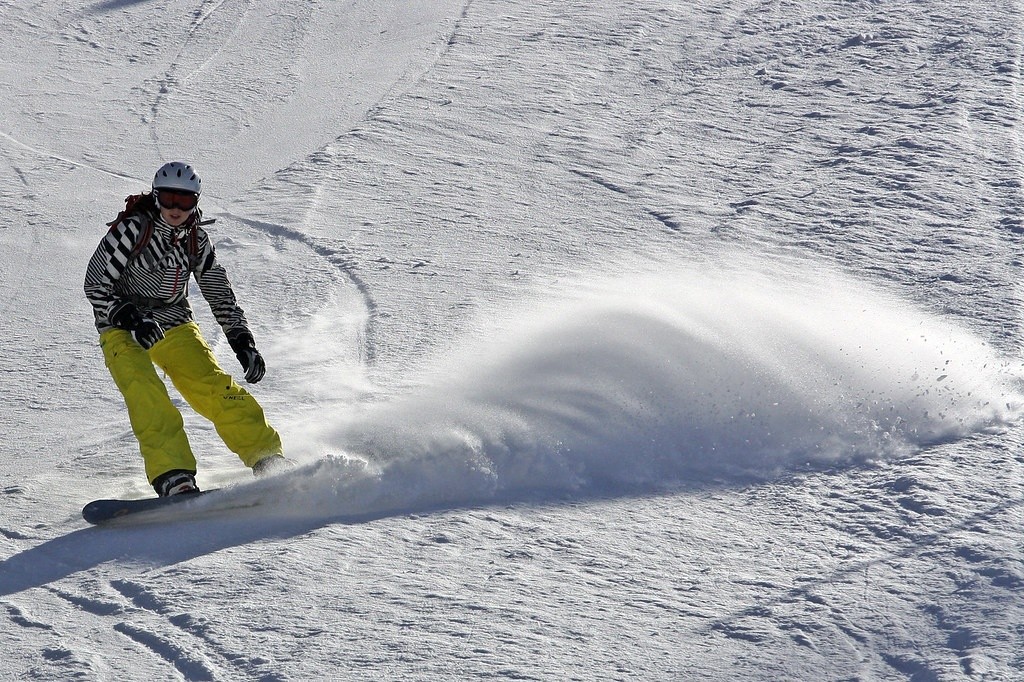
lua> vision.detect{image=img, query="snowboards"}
[81,468,385,531]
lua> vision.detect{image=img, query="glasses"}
[157,191,198,211]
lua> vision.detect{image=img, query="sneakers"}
[253,455,296,478]
[156,472,193,495]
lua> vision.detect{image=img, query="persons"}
[83,161,298,498]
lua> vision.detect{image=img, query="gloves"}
[236,346,266,384]
[108,301,165,350]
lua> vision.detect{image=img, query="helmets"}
[152,162,202,194]
